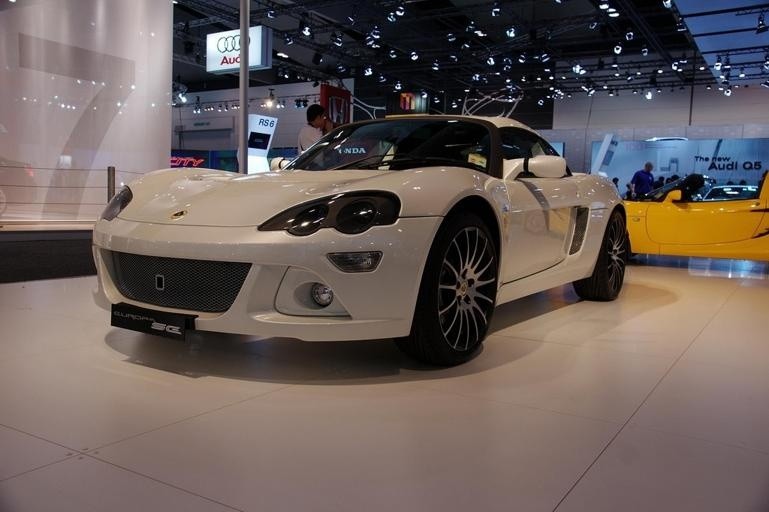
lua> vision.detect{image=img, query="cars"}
[623,170,769,261]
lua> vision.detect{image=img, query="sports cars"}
[91,114,626,367]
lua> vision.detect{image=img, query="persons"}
[297,104,334,157]
[612,162,747,200]
